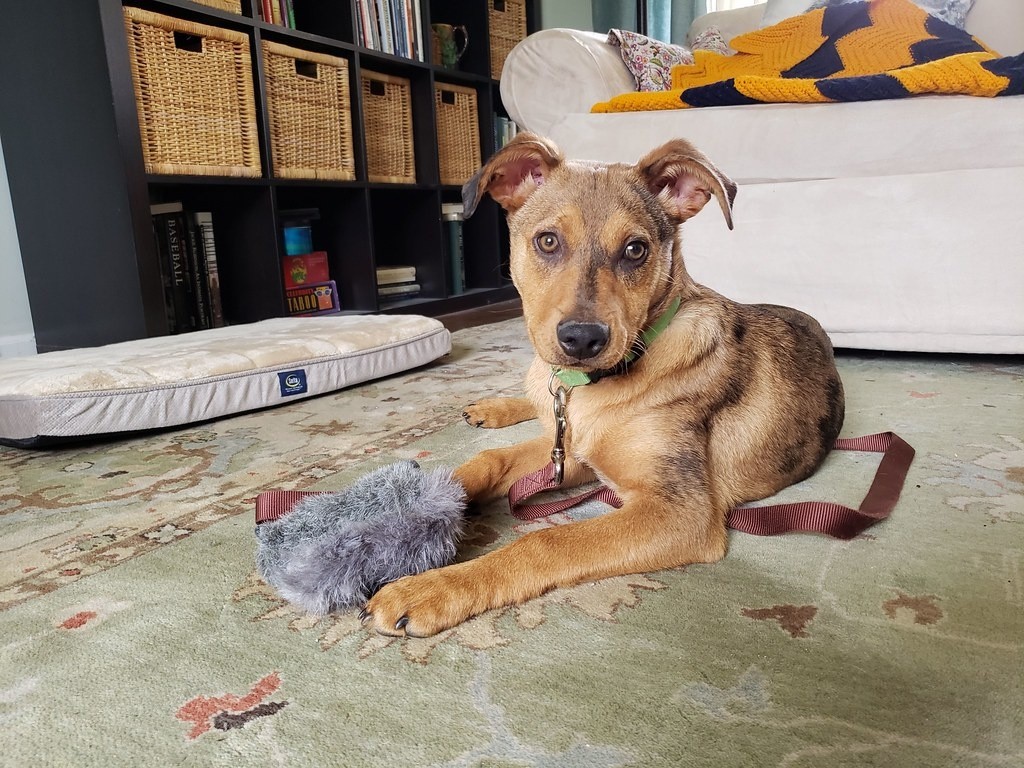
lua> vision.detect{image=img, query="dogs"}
[354,130,847,642]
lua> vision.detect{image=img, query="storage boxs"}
[284,252,330,289]
[285,280,340,318]
[489,0,527,79]
[433,81,482,184]
[123,6,263,178]
[260,40,358,182]
[360,67,416,184]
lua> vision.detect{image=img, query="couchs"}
[500,0,1024,354]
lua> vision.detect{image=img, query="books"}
[260,0,296,30]
[151,202,224,334]
[356,0,424,62]
[377,266,421,304]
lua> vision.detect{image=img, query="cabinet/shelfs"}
[0,0,544,355]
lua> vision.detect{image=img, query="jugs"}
[432,23,469,69]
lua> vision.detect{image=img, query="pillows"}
[605,27,730,93]
